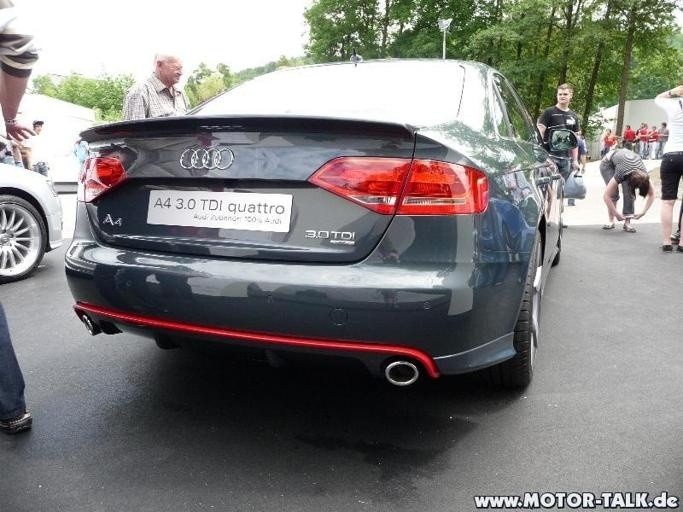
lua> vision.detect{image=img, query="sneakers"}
[671,230,681,241]
[623,223,636,233]
[662,241,674,251]
[601,223,615,229]
[677,244,683,252]
[0,412,34,434]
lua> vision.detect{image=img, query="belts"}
[665,151,683,156]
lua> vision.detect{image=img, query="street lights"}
[436,15,452,58]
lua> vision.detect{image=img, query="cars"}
[0,161,63,284]
[61,58,580,393]
